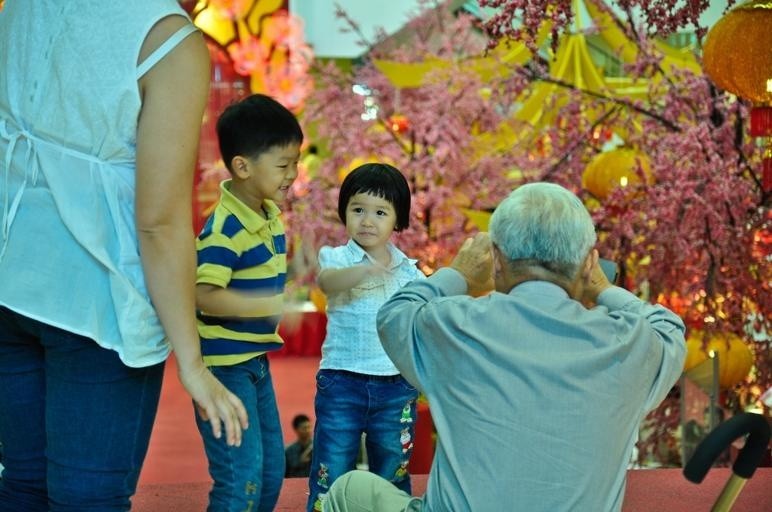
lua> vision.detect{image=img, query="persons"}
[1,0,252,509]
[303,162,429,512]
[191,93,306,509]
[318,179,689,512]
[285,414,313,479]
